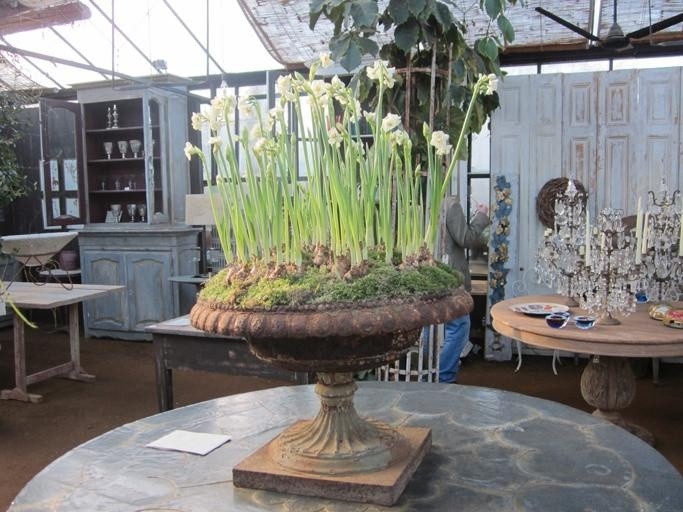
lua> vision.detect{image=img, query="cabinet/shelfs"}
[38,72,192,228]
[75,225,208,345]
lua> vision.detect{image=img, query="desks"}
[487,295,682,448]
[38,266,84,337]
[166,275,206,319]
[7,377,681,512]
[141,315,314,413]
[0,279,127,404]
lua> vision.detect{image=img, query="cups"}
[59,248,78,271]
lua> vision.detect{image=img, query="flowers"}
[179,49,500,296]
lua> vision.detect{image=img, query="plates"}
[509,302,570,314]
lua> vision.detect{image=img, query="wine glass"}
[110,202,146,224]
[101,139,156,160]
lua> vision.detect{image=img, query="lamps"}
[183,193,223,278]
[527,178,682,329]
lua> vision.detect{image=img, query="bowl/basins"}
[635,291,650,305]
[544,311,571,330]
[0,231,78,266]
[574,316,598,329]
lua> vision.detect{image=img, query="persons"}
[423,192,492,384]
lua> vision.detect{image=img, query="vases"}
[188,287,476,475]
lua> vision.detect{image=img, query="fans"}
[530,0,681,59]
